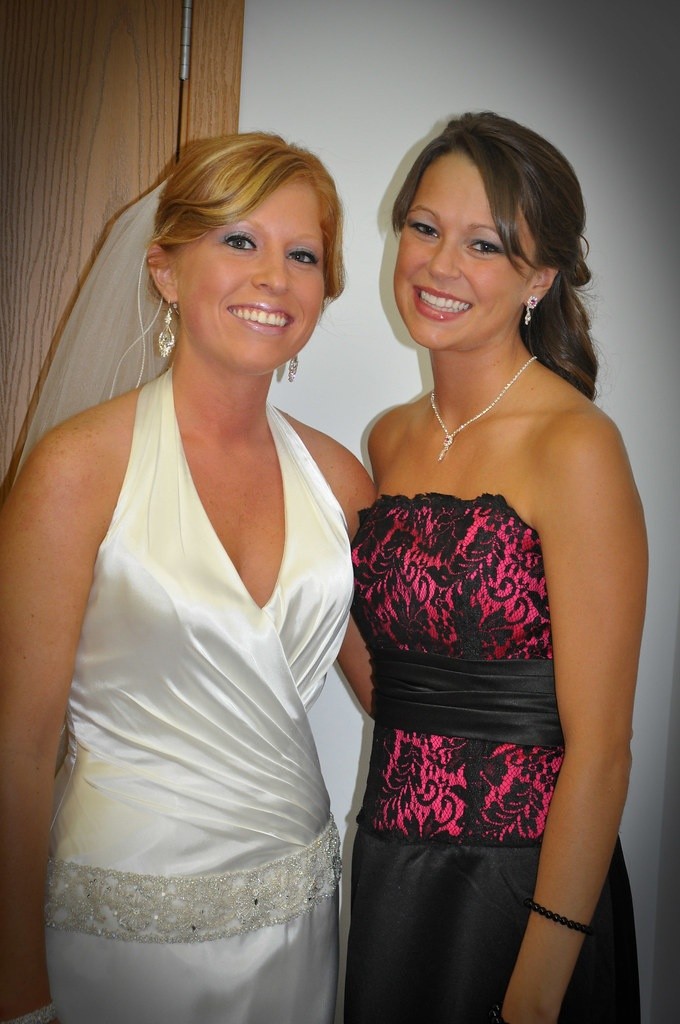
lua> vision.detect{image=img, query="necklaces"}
[429,357,537,462]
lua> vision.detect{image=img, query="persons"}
[0,130,375,1023]
[342,111,649,1024]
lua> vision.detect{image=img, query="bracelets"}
[523,899,592,936]
[10,1003,56,1024]
